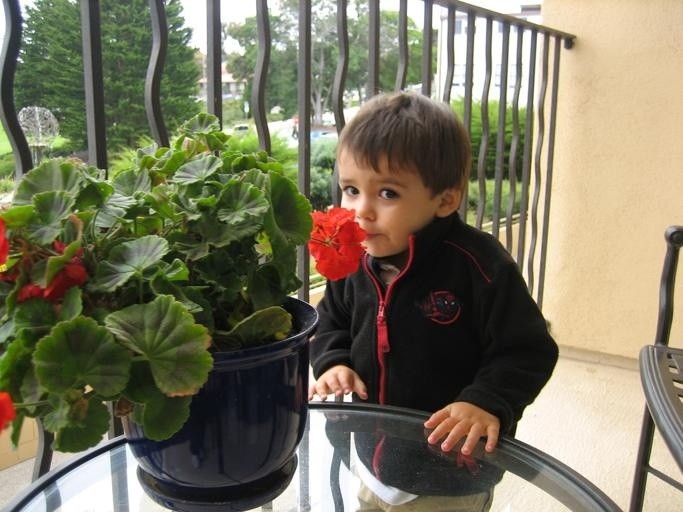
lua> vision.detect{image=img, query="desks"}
[0,400,622,512]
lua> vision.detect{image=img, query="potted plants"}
[117,296,320,511]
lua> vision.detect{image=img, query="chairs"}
[629,226,683,510]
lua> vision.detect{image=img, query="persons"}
[303,88,561,512]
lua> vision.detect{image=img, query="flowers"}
[0,113,369,453]
